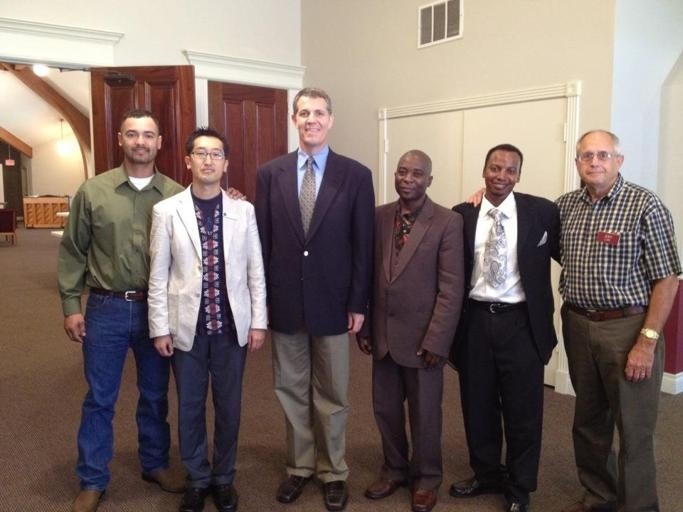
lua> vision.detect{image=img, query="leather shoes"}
[141,470,237,512]
[72,490,101,512]
[562,500,587,512]
[276,474,527,512]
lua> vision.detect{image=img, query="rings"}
[640,373,645,376]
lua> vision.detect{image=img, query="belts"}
[565,302,647,321]
[467,298,523,314]
[90,287,147,302]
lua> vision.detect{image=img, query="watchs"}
[639,326,660,341]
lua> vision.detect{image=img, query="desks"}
[51,211,69,239]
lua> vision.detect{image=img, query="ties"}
[299,158,316,240]
[483,209,507,289]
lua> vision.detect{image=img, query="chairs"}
[0,207,18,246]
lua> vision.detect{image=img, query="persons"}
[57,109,248,512]
[452,143,564,512]
[147,125,269,512]
[356,149,466,512]
[466,130,683,511]
[254,86,374,512]
